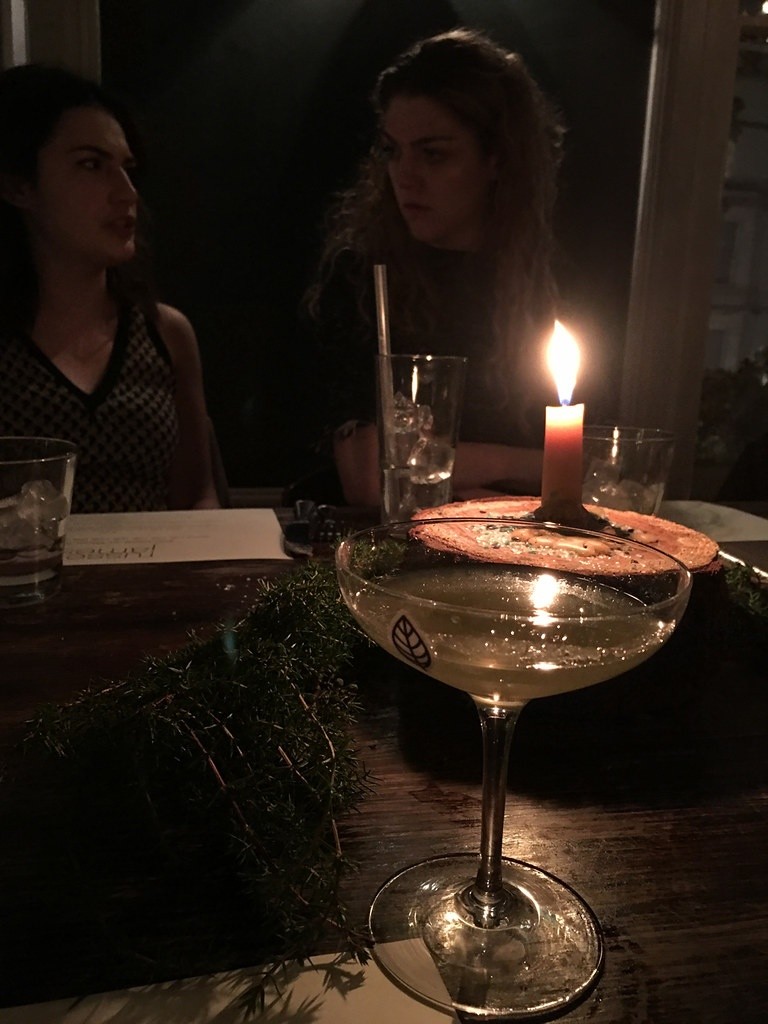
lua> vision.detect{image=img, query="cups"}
[378,353,466,524]
[583,425,678,516]
[1,436,78,610]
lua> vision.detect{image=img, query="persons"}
[304,28,604,507]
[0,62,223,513]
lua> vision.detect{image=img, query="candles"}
[542,317,585,517]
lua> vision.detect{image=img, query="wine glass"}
[334,519,691,1020]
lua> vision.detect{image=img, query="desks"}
[0,501,768,1024]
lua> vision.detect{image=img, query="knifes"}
[285,501,314,567]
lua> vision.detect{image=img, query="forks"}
[309,505,342,542]
